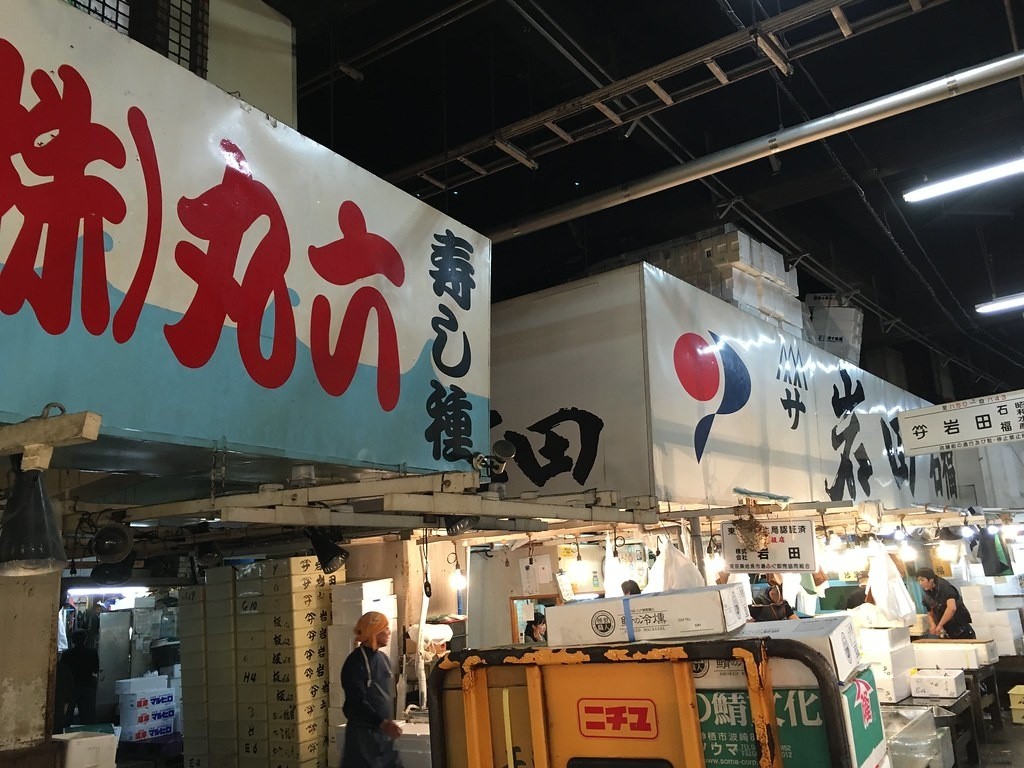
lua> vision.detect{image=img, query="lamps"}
[302,525,349,574]
[91,562,132,587]
[0,454,69,577]
[196,526,223,565]
[471,439,516,474]
[88,524,132,563]
[444,514,480,536]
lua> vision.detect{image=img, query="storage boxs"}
[52,555,468,768]
[545,538,1023,768]
[586,224,865,366]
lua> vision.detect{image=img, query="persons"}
[844,571,869,610]
[752,583,800,619]
[61,629,100,725]
[67,611,75,631]
[524,612,546,643]
[621,580,641,596]
[915,566,976,639]
[340,611,405,768]
[80,604,102,648]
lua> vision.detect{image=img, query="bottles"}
[592,570,600,590]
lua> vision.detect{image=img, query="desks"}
[964,665,1004,744]
[897,690,982,768]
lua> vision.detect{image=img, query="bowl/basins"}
[750,603,785,620]
